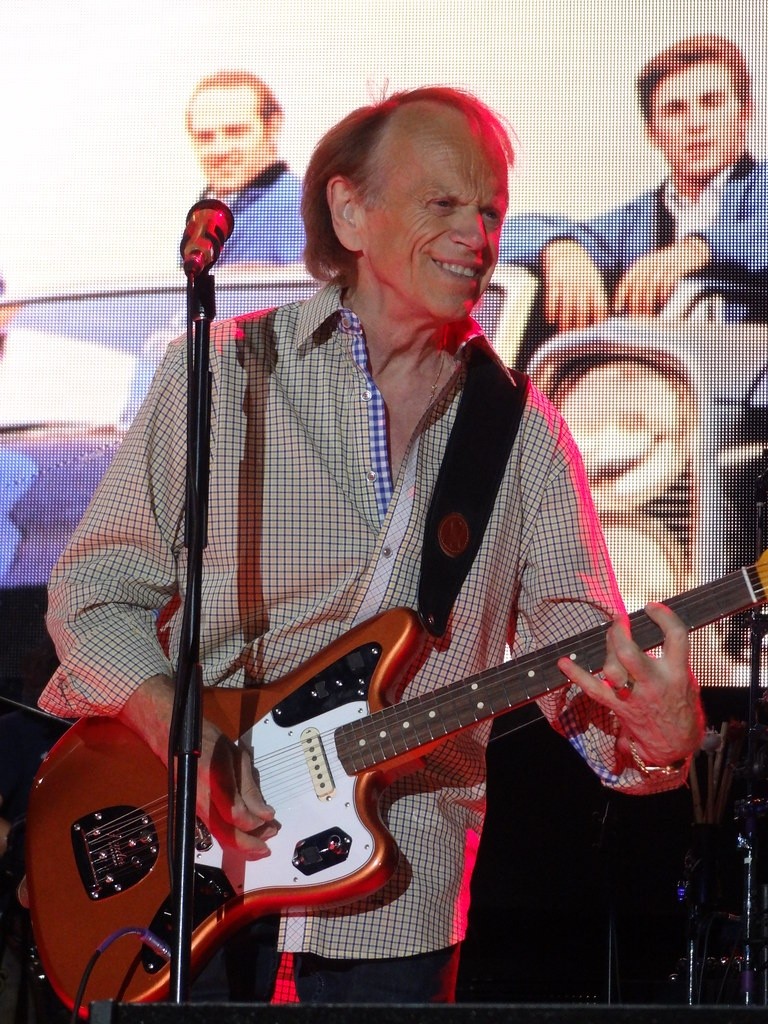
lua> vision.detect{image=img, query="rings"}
[611,672,636,699]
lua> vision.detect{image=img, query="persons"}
[498,34,767,334]
[180,70,310,268]
[37,86,708,1005]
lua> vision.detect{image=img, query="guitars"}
[25,550,768,1020]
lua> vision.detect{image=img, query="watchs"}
[629,738,693,782]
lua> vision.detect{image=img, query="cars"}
[0,249,768,688]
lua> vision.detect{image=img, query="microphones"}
[180,200,234,274]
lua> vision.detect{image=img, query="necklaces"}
[427,350,446,409]
